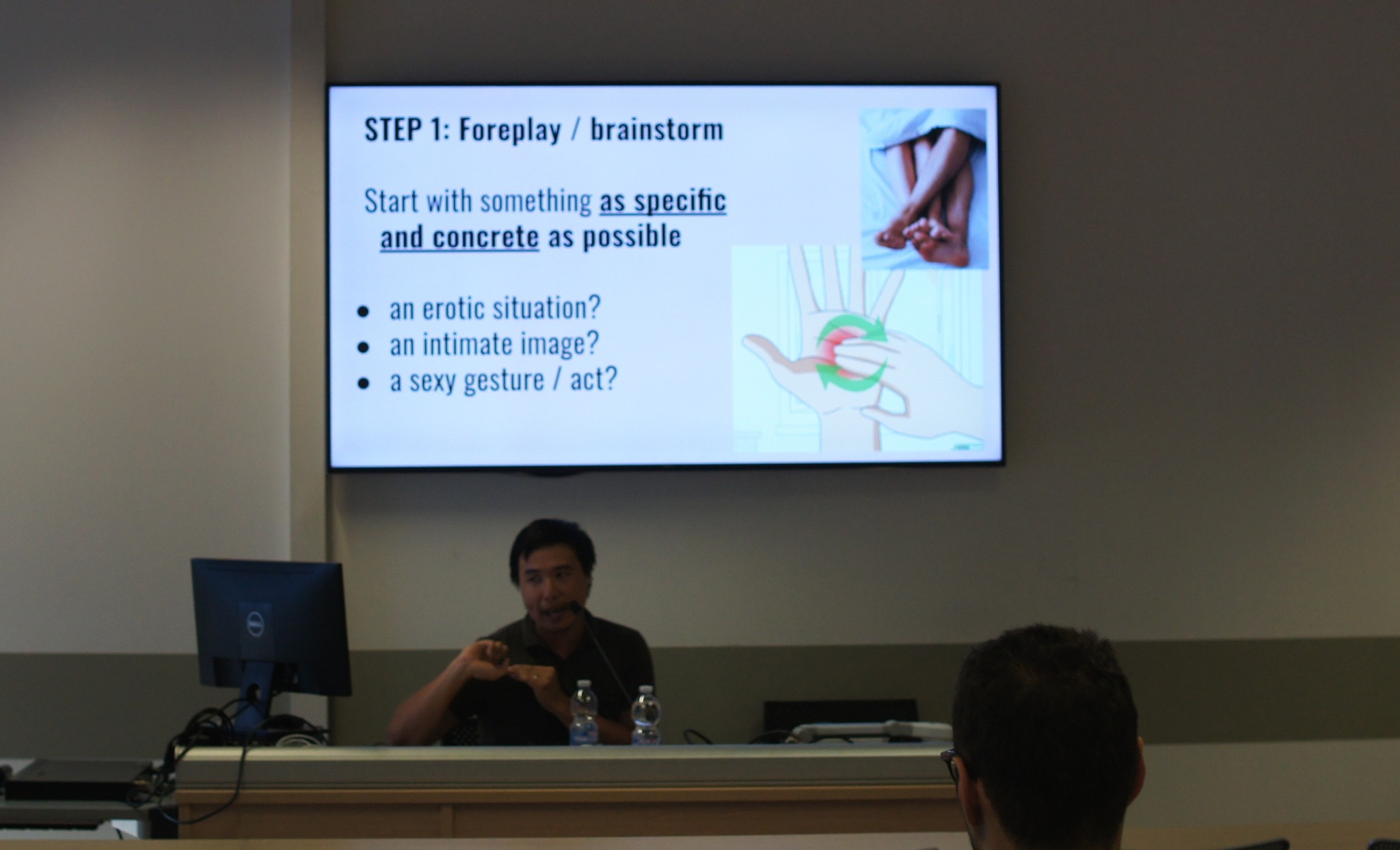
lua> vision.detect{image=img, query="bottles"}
[631,685,662,744]
[569,680,599,745]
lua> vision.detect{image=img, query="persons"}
[874,128,979,270]
[942,626,1145,849]
[388,517,656,745]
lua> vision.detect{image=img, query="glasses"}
[940,748,971,780]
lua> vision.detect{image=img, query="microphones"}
[568,603,632,710]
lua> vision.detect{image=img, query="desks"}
[178,746,964,840]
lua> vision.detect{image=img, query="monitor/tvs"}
[188,555,351,746]
